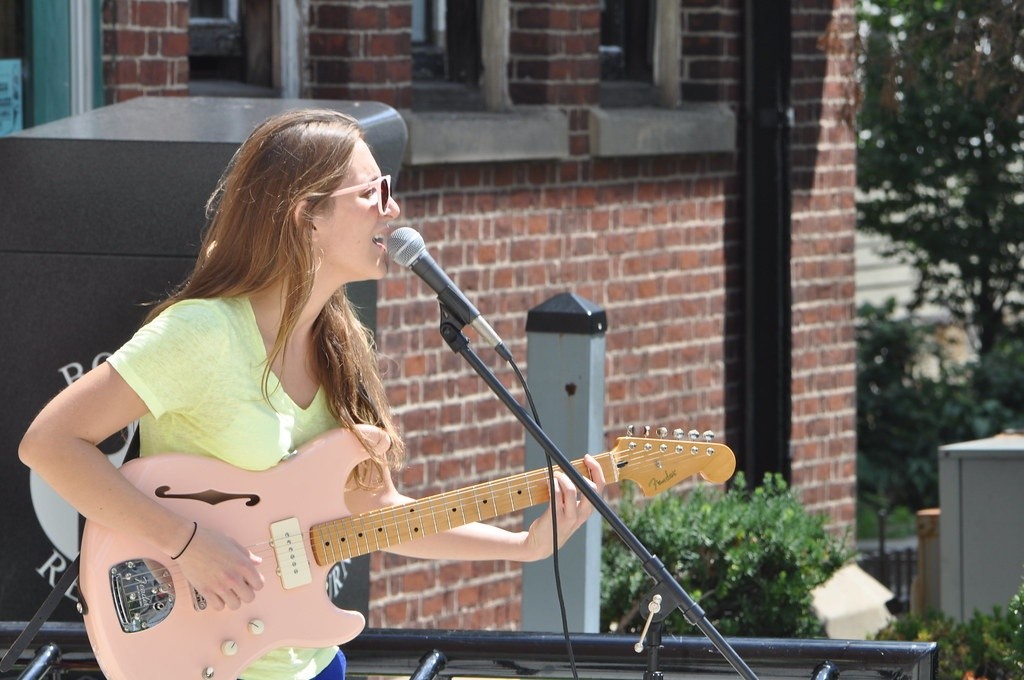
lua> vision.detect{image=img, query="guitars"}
[77,424,736,680]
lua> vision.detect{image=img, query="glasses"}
[305,175,391,216]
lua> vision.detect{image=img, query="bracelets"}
[171,522,197,560]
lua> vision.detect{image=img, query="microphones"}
[387,227,514,362]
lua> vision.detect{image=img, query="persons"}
[18,108,606,680]
